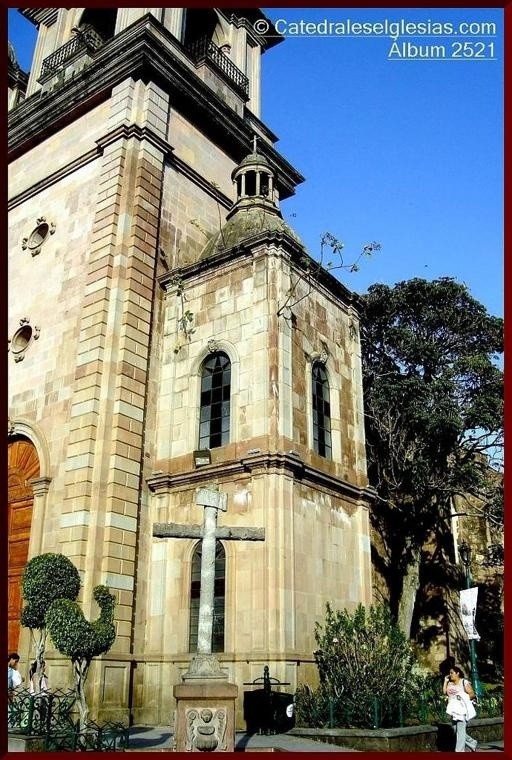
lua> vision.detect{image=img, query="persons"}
[29,659,56,731]
[7,652,21,727]
[10,662,26,727]
[442,665,478,752]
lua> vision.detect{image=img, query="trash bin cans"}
[243,666,295,735]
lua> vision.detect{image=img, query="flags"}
[459,586,482,643]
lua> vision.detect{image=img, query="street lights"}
[458,541,483,702]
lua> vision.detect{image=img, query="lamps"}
[193,450,211,468]
